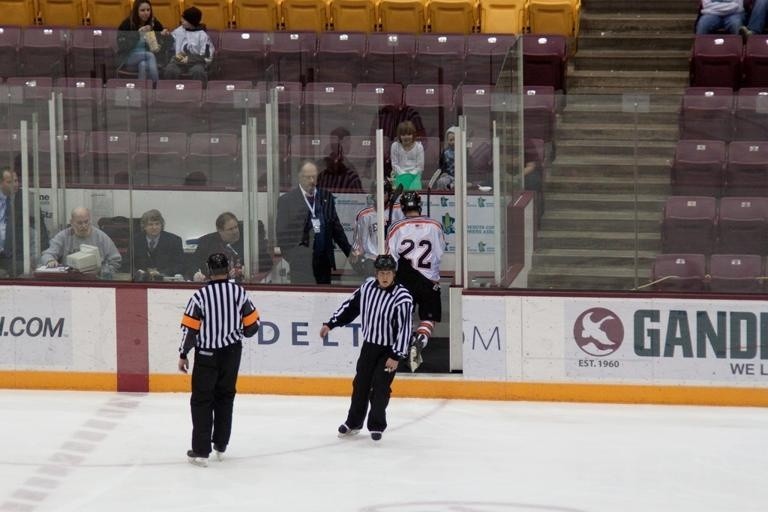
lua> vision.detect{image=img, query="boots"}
[412,320,435,369]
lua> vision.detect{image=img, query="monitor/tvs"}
[66,244,101,273]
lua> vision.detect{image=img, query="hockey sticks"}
[389,183,402,227]
[426,169,443,218]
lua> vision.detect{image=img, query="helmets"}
[399,191,422,211]
[370,178,391,203]
[205,254,230,277]
[374,253,396,277]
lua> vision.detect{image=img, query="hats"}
[183,7,202,29]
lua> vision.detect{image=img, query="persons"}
[349,179,406,284]
[320,254,415,441]
[160,7,216,89]
[485,119,543,193]
[737,0,768,45]
[186,213,272,283]
[39,206,122,277]
[435,126,473,193]
[0,166,49,279]
[177,253,260,468]
[370,81,424,174]
[315,143,363,194]
[276,161,352,286]
[116,0,174,89]
[388,122,425,192]
[385,192,446,375]
[125,209,185,280]
[695,0,744,34]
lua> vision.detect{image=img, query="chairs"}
[0,1,582,273]
[652,32,768,297]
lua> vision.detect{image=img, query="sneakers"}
[338,422,351,434]
[371,432,382,441]
[214,446,227,452]
[187,450,209,457]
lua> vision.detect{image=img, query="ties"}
[4,196,11,223]
[149,239,154,260]
